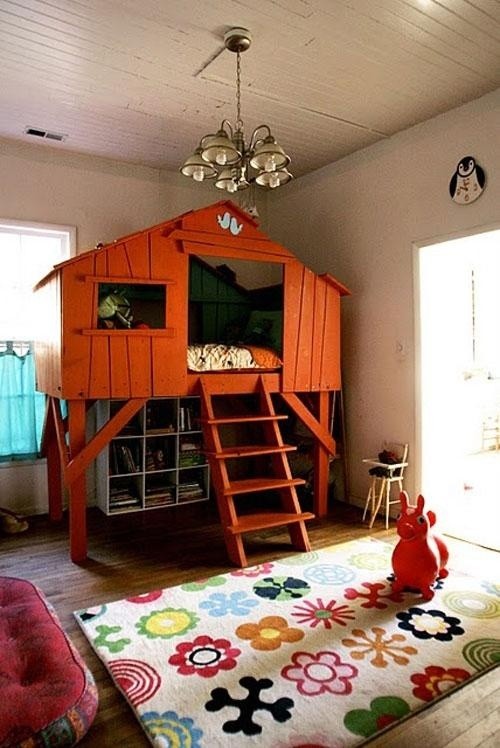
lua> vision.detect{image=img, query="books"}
[108,407,204,511]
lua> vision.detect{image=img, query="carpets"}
[67,532,499,746]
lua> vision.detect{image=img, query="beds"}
[26,197,350,573]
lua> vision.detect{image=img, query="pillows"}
[237,325,273,348]
[248,307,282,349]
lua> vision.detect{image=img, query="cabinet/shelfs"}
[92,391,216,517]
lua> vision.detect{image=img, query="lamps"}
[177,26,295,196]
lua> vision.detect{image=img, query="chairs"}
[356,437,411,531]
